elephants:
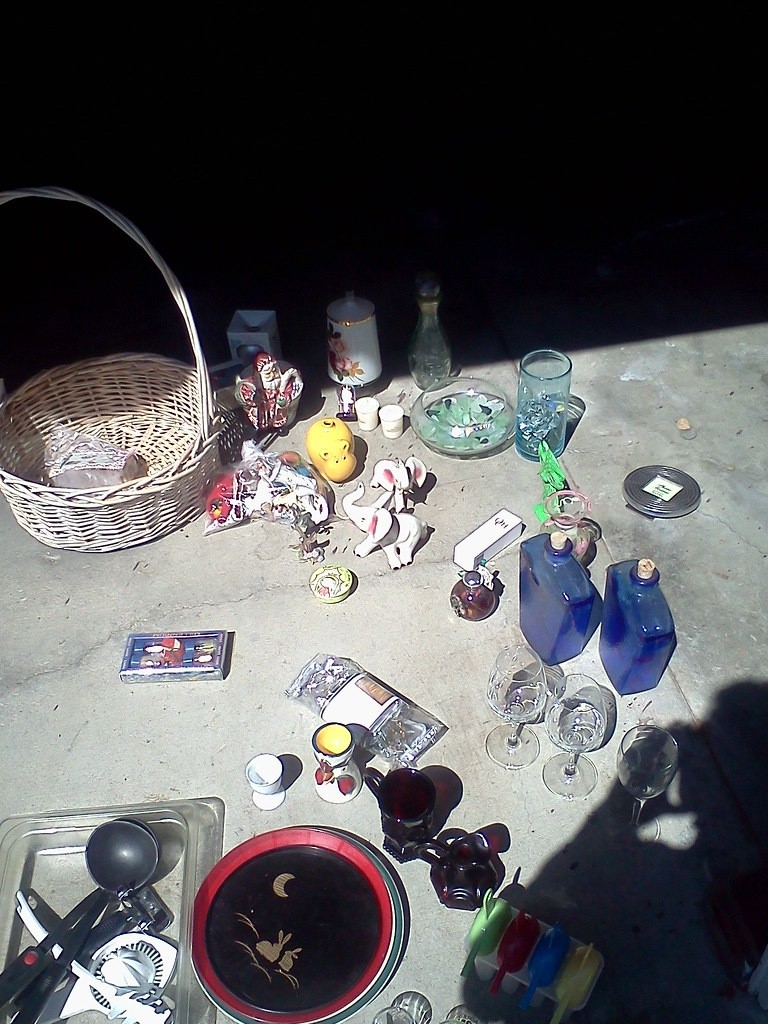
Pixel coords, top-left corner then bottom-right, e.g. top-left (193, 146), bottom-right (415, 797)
top-left (332, 457), bottom-right (428, 570)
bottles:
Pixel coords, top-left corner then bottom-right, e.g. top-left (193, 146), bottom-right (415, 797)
top-left (518, 531), bottom-right (594, 667)
top-left (408, 281), bottom-right (452, 390)
top-left (599, 558), bottom-right (674, 696)
top-left (540, 491), bottom-right (590, 563)
top-left (449, 571), bottom-right (495, 621)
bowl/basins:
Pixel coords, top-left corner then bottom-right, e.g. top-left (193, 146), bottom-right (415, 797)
top-left (410, 377), bottom-right (515, 455)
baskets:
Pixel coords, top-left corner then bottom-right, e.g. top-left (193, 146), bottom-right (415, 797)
top-left (0, 182), bottom-right (223, 553)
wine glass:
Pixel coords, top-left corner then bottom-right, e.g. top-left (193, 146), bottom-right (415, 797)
top-left (542, 673), bottom-right (607, 797)
top-left (485, 644), bottom-right (547, 769)
top-left (616, 725), bottom-right (678, 847)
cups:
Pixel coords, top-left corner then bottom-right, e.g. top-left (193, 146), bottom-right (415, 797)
top-left (245, 753), bottom-right (283, 795)
top-left (379, 404), bottom-right (405, 439)
top-left (327, 291), bottom-right (382, 388)
top-left (373, 1007), bottom-right (415, 1024)
top-left (515, 349), bottom-right (572, 461)
top-left (440, 1020), bottom-right (463, 1024)
top-left (410, 833), bottom-right (496, 911)
top-left (362, 767), bottom-right (438, 864)
top-left (355, 397), bottom-right (379, 430)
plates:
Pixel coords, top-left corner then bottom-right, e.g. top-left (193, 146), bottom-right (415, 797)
top-left (187, 825), bottom-right (404, 1024)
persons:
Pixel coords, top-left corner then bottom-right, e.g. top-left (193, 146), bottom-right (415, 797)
top-left (237, 353), bottom-right (298, 437)
